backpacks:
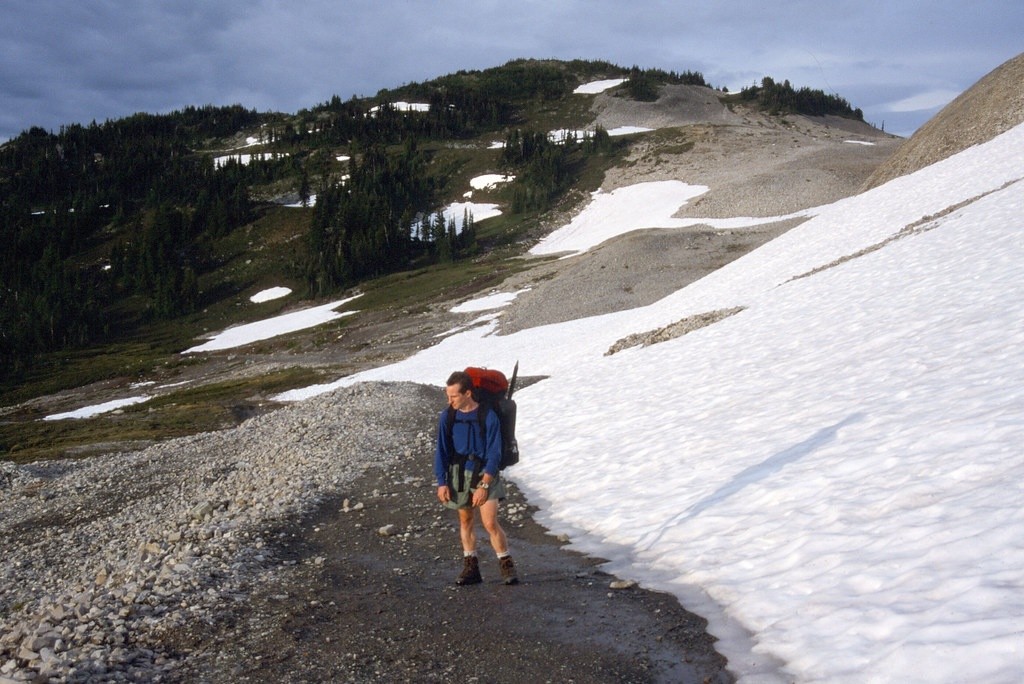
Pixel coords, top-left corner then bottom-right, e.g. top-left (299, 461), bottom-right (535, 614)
top-left (447, 367), bottom-right (519, 489)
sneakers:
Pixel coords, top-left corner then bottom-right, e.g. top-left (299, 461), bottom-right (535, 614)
top-left (499, 555), bottom-right (520, 585)
top-left (455, 556), bottom-right (483, 584)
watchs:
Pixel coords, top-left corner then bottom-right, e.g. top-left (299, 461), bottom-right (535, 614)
top-left (478, 481), bottom-right (490, 490)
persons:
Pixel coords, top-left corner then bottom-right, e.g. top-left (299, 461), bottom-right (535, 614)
top-left (433, 370), bottom-right (519, 589)
